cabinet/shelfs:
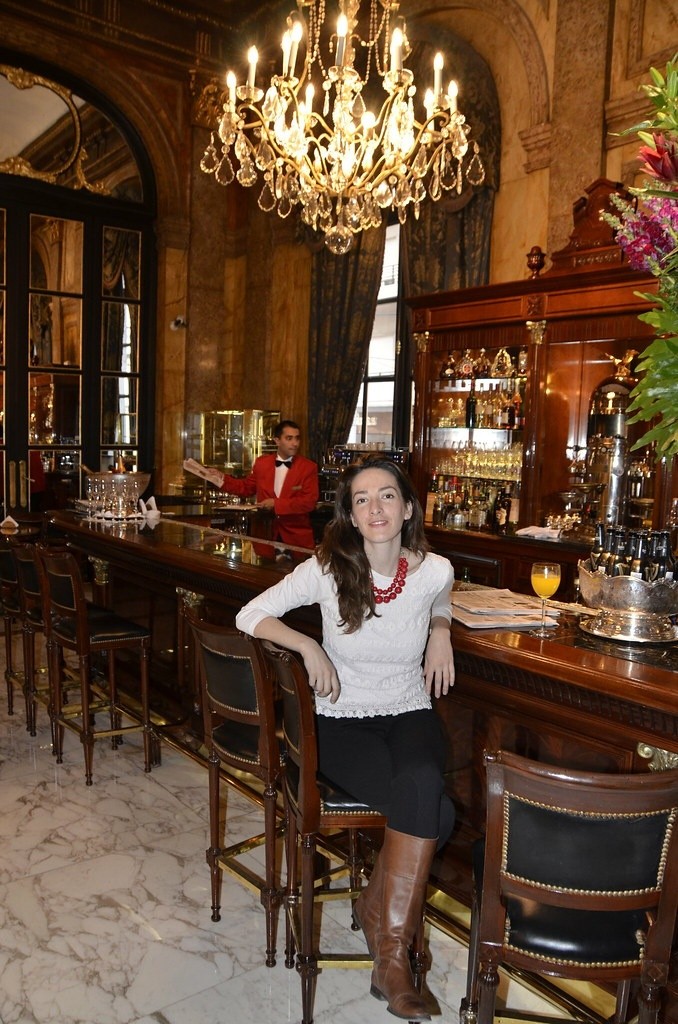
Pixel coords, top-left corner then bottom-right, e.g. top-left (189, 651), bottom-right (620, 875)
top-left (402, 259), bottom-right (678, 603)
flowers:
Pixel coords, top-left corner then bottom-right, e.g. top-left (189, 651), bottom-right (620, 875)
top-left (594, 55), bottom-right (677, 455)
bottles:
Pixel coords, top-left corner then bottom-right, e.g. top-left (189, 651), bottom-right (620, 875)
top-left (459, 568), bottom-right (471, 584)
top-left (590, 521), bottom-right (676, 586)
top-left (428, 348), bottom-right (531, 534)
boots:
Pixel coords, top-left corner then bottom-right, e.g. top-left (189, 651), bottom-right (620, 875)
top-left (370, 823), bottom-right (441, 1021)
top-left (353, 843), bottom-right (387, 961)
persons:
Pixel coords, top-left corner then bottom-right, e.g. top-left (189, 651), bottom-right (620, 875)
top-left (207, 420), bottom-right (318, 513)
top-left (235, 454), bottom-right (456, 1024)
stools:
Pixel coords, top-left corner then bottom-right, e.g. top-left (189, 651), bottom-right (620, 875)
top-left (1, 527), bottom-right (678, 1023)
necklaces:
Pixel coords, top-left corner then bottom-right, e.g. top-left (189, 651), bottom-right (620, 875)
top-left (370, 547), bottom-right (406, 603)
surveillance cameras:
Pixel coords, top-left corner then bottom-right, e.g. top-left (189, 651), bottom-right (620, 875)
top-left (170, 318), bottom-right (183, 331)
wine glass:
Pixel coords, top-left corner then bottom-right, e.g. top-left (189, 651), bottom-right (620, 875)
top-left (558, 481), bottom-right (654, 533)
top-left (85, 476), bottom-right (140, 523)
top-left (531, 561), bottom-right (561, 638)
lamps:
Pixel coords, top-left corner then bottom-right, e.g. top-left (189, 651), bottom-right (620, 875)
top-left (186, 0), bottom-right (485, 256)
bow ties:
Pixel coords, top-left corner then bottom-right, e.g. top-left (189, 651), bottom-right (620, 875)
top-left (275, 460), bottom-right (293, 468)
